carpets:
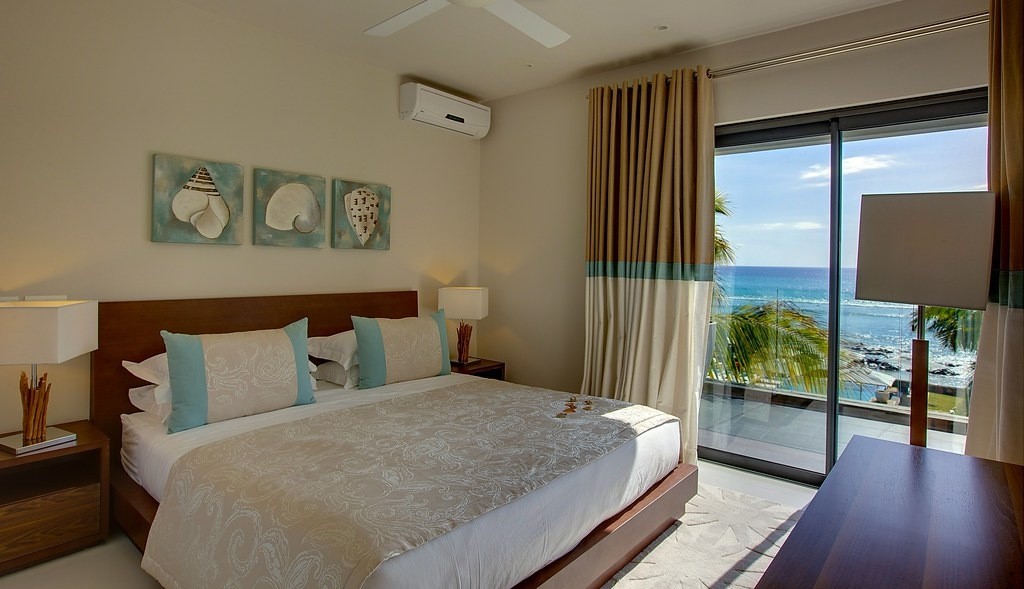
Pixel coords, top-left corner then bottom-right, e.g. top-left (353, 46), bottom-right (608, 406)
top-left (598, 483), bottom-right (807, 589)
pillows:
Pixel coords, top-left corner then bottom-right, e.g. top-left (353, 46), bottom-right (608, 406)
top-left (307, 309), bottom-right (452, 391)
top-left (122, 314), bottom-right (317, 435)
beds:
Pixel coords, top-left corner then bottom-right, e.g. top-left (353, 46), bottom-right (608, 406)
top-left (89, 290), bottom-right (698, 589)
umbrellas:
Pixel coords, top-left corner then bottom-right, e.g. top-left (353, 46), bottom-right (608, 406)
top-left (757, 358), bottom-right (812, 376)
top-left (842, 366), bottom-right (895, 399)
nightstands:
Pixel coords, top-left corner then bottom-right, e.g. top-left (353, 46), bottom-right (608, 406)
top-left (0, 419), bottom-right (111, 577)
top-left (449, 356), bottom-right (506, 381)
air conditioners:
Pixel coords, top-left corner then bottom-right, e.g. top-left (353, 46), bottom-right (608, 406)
top-left (399, 82), bottom-right (496, 140)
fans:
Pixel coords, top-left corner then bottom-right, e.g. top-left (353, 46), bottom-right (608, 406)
top-left (363, 0), bottom-right (573, 48)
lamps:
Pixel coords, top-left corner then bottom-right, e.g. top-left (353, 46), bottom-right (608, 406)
top-left (438, 286), bottom-right (489, 368)
top-left (0, 299), bottom-right (99, 456)
top-left (855, 190), bottom-right (999, 449)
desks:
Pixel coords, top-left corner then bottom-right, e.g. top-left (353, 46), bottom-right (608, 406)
top-left (753, 435), bottom-right (1024, 589)
